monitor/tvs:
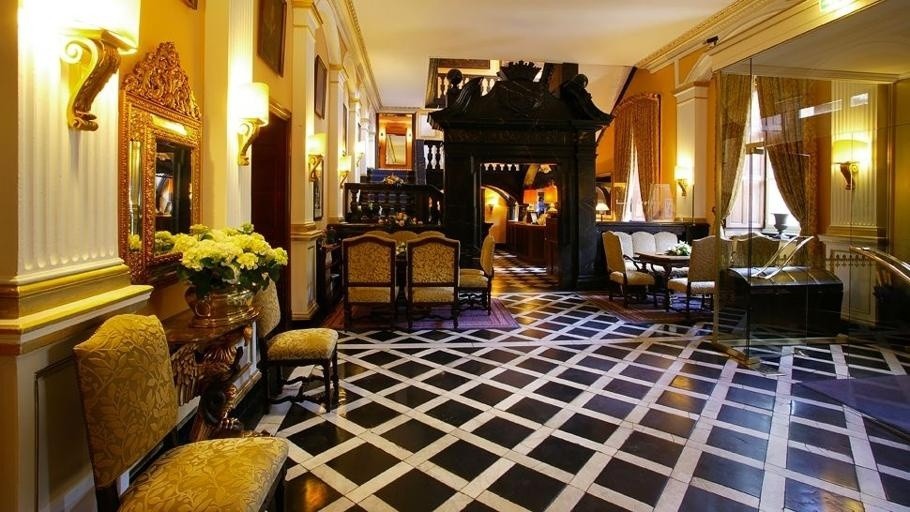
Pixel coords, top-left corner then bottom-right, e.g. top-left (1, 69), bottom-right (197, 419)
top-left (528, 211), bottom-right (539, 223)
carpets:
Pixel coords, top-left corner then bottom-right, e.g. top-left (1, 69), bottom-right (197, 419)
top-left (319, 297), bottom-right (521, 331)
top-left (797, 372), bottom-right (909, 443)
top-left (573, 290), bottom-right (714, 325)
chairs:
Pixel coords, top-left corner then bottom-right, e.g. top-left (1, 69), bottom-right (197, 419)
top-left (340, 229), bottom-right (496, 330)
top-left (251, 272), bottom-right (340, 415)
top-left (70, 312), bottom-right (291, 512)
top-left (601, 230), bottom-right (825, 324)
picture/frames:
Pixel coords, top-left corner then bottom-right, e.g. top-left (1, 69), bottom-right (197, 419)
top-left (314, 54), bottom-right (327, 120)
top-left (344, 104), bottom-right (349, 153)
top-left (313, 158), bottom-right (325, 221)
top-left (184, 0), bottom-right (198, 9)
top-left (257, 0), bottom-right (288, 78)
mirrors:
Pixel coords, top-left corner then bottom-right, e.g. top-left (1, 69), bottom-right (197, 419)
top-left (117, 39), bottom-right (204, 288)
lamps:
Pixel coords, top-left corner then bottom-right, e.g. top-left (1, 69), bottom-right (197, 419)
top-left (834, 139), bottom-right (869, 191)
top-left (238, 81), bottom-right (271, 167)
top-left (613, 182), bottom-right (628, 205)
top-left (675, 165), bottom-right (690, 197)
top-left (308, 133), bottom-right (325, 182)
top-left (339, 155), bottom-right (352, 188)
top-left (488, 203), bottom-right (495, 214)
top-left (61, 0), bottom-right (144, 128)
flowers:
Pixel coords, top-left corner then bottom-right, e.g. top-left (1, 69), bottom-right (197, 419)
top-left (378, 212), bottom-right (425, 228)
top-left (381, 173), bottom-right (408, 187)
top-left (171, 222), bottom-right (289, 299)
top-left (128, 229), bottom-right (180, 255)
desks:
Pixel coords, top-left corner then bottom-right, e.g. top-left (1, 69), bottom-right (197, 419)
top-left (160, 308), bottom-right (274, 442)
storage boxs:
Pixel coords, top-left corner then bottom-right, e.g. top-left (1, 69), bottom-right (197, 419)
top-left (717, 266), bottom-right (844, 339)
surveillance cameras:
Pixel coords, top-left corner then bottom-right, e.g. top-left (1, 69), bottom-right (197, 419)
top-left (703, 35), bottom-right (719, 48)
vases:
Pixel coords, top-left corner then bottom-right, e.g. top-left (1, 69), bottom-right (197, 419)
top-left (184, 284), bottom-right (260, 329)
